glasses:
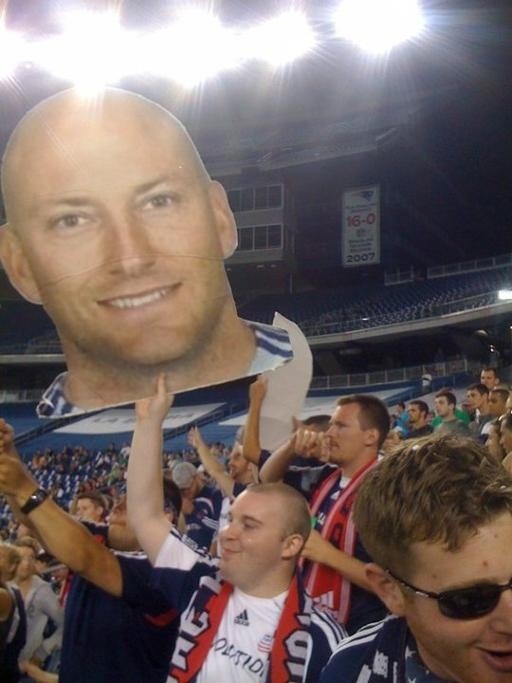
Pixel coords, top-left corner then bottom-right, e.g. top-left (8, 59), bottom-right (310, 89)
top-left (386, 570), bottom-right (512, 622)
top-left (487, 398), bottom-right (497, 403)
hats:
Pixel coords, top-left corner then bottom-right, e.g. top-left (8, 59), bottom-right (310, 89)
top-left (173, 462), bottom-right (198, 491)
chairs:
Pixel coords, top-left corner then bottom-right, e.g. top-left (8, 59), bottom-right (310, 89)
top-left (0, 376), bottom-right (421, 508)
top-left (236, 266), bottom-right (512, 348)
top-left (1, 298), bottom-right (61, 354)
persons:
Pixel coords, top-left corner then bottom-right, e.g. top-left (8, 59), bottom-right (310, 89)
top-left (2, 323), bottom-right (512, 683)
top-left (0, 83), bottom-right (295, 419)
